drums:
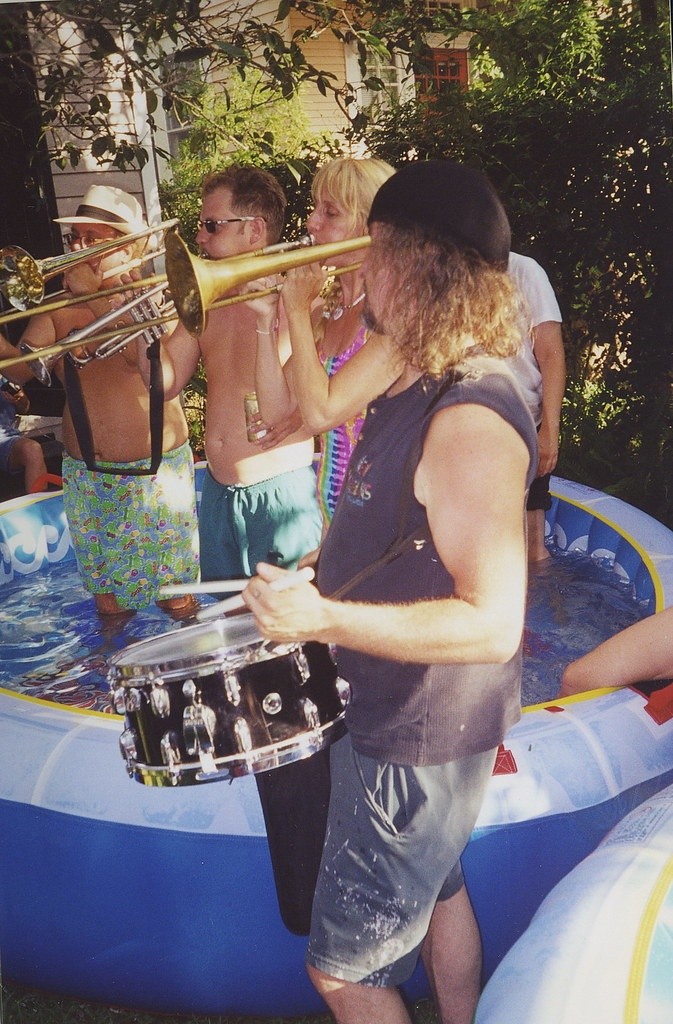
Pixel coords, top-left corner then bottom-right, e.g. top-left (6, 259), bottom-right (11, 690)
top-left (105, 612), bottom-right (352, 787)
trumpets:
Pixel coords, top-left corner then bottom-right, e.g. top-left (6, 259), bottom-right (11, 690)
top-left (1, 216), bottom-right (375, 386)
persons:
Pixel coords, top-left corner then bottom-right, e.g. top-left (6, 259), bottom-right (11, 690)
top-left (0, 185), bottom-right (202, 613)
top-left (104, 160), bottom-right (328, 620)
top-left (243, 159), bottom-right (397, 534)
top-left (505, 252), bottom-right (566, 561)
top-left (243, 158), bottom-right (541, 1024)
top-left (0, 374), bottom-right (48, 496)
top-left (560, 607), bottom-right (673, 695)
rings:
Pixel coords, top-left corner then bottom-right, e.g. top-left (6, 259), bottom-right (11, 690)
top-left (254, 584), bottom-right (267, 598)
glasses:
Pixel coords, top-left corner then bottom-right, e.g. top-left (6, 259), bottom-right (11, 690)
top-left (64, 234), bottom-right (115, 251)
top-left (197, 217), bottom-right (268, 234)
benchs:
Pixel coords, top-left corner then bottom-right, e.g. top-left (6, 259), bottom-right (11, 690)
top-left (0, 440), bottom-right (64, 503)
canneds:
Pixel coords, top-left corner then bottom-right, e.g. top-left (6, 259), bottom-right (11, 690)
top-left (245, 392), bottom-right (271, 441)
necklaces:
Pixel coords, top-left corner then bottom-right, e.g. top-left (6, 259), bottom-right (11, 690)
top-left (332, 292), bottom-right (366, 321)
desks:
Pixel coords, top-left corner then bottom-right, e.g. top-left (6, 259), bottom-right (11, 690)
top-left (16, 414), bottom-right (63, 444)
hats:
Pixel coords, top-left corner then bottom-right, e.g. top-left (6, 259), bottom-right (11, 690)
top-left (366, 161), bottom-right (511, 272)
top-left (53, 184), bottom-right (157, 252)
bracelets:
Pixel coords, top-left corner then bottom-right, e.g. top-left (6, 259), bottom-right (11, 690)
top-left (256, 330), bottom-right (271, 334)
